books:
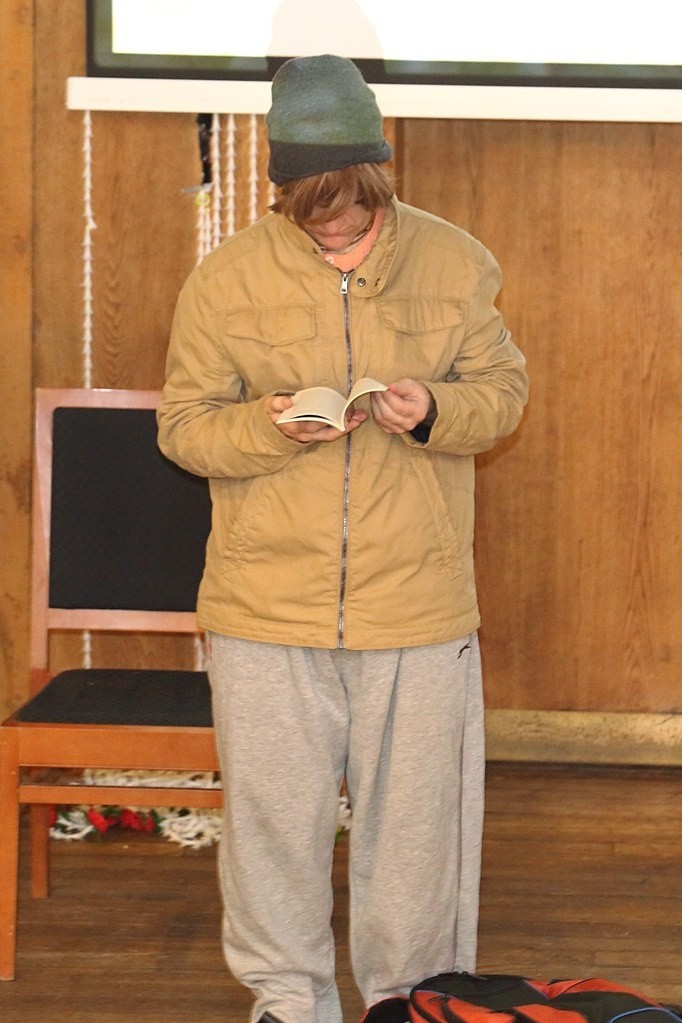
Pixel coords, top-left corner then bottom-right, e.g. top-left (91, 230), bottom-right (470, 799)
top-left (275, 376), bottom-right (390, 432)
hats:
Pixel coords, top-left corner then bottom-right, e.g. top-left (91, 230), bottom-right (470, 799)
top-left (265, 54), bottom-right (393, 184)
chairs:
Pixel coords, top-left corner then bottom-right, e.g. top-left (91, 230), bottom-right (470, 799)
top-left (0, 390), bottom-right (224, 981)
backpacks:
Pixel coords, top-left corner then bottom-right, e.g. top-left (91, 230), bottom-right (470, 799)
top-left (356, 970), bottom-right (682, 1023)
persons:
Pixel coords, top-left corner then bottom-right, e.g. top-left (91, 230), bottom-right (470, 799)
top-left (156, 54), bottom-right (529, 1023)
